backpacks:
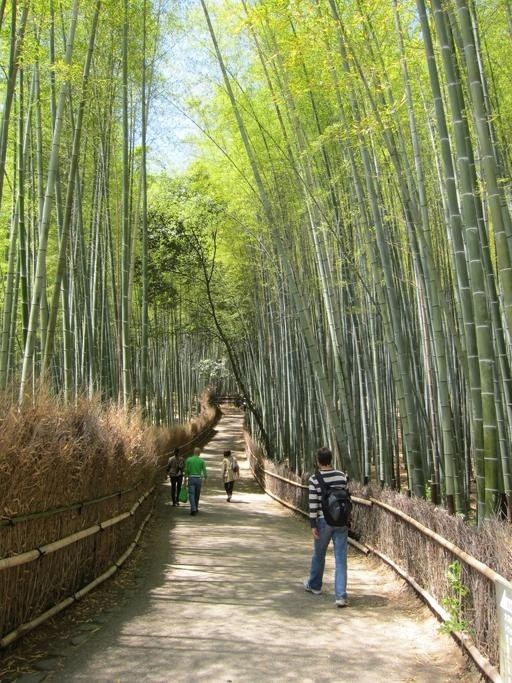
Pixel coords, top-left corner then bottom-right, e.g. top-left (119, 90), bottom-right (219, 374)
top-left (314, 469), bottom-right (353, 528)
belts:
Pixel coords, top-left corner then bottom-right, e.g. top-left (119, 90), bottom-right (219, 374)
top-left (188, 474), bottom-right (201, 478)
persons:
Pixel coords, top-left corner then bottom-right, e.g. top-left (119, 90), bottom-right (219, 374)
top-left (303, 445), bottom-right (351, 608)
top-left (184, 446), bottom-right (208, 515)
top-left (221, 448), bottom-right (239, 502)
top-left (164, 447), bottom-right (185, 507)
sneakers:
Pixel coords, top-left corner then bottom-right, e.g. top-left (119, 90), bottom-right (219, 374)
top-left (190, 509), bottom-right (199, 515)
top-left (334, 597), bottom-right (349, 607)
top-left (227, 495), bottom-right (232, 502)
top-left (302, 578), bottom-right (323, 594)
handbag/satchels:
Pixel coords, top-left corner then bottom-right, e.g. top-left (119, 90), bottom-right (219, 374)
top-left (178, 476), bottom-right (188, 503)
top-left (231, 457), bottom-right (239, 473)
